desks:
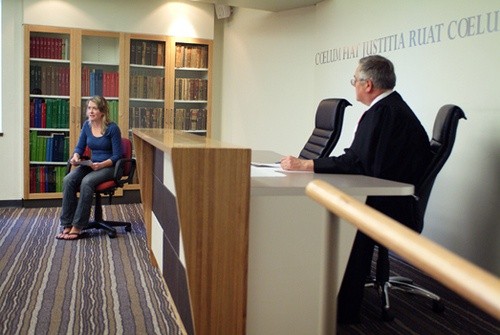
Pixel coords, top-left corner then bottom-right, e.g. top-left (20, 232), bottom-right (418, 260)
top-left (245, 151), bottom-right (414, 335)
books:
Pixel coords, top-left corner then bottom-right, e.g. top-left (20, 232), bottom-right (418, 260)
top-left (29, 36), bottom-right (70, 193)
top-left (81, 65), bottom-right (119, 126)
top-left (175, 44), bottom-right (208, 130)
top-left (129, 41), bottom-right (164, 158)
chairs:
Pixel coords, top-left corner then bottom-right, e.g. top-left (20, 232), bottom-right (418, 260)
top-left (365, 104), bottom-right (468, 324)
top-left (66, 138), bottom-right (136, 239)
top-left (296, 98), bottom-right (352, 160)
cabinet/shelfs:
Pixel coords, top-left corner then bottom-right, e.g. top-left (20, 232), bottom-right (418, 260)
top-left (22, 23), bottom-right (214, 199)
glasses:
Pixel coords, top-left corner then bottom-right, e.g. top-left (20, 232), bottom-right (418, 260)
top-left (350, 78), bottom-right (367, 87)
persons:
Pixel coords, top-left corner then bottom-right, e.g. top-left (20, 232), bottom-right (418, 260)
top-left (56, 95), bottom-right (125, 241)
top-left (280, 55), bottom-right (430, 323)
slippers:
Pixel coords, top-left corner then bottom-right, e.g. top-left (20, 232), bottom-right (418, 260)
top-left (55, 229), bottom-right (70, 240)
top-left (66, 231), bottom-right (81, 241)
